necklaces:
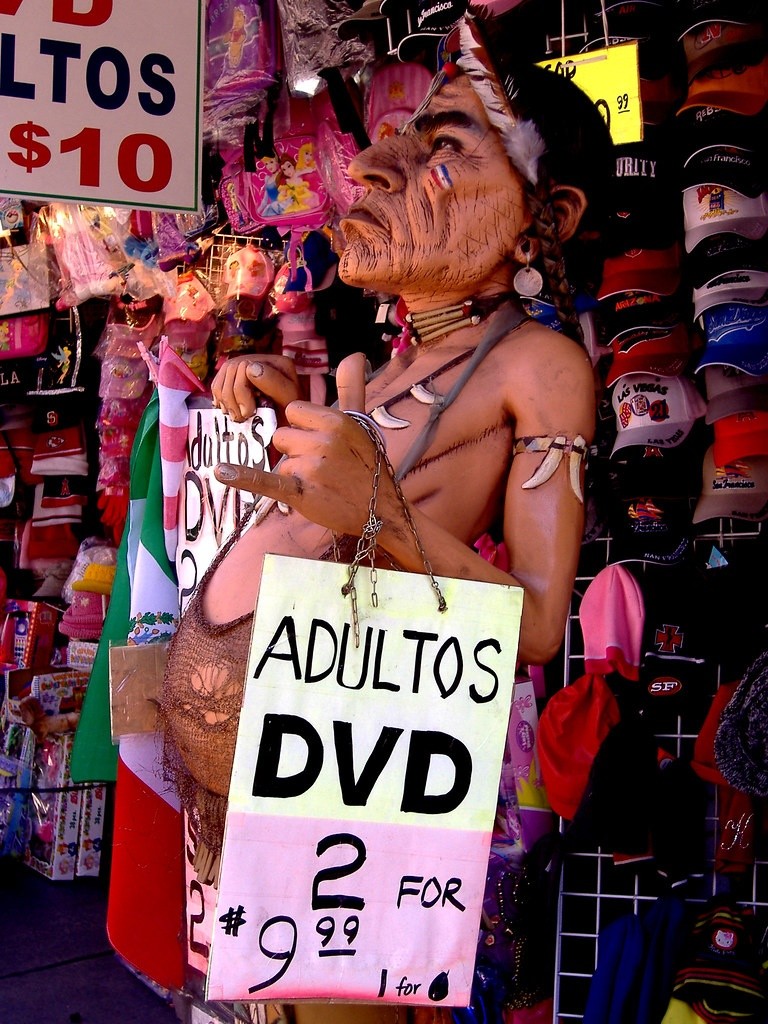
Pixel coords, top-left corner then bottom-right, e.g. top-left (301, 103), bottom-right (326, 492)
top-left (407, 297), bottom-right (486, 349)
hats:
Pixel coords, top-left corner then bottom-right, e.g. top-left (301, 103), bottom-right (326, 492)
top-left (342, 0), bottom-right (767, 1023)
top-left (0, 202), bottom-right (326, 640)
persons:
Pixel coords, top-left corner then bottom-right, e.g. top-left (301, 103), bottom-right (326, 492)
top-left (160, 57), bottom-right (616, 1024)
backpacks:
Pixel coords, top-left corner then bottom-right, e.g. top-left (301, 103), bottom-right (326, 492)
top-left (203, 0), bottom-right (277, 104)
top-left (218, 84), bottom-right (367, 234)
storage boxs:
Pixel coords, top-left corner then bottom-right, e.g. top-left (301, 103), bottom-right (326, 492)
top-left (0, 601), bottom-right (106, 881)
